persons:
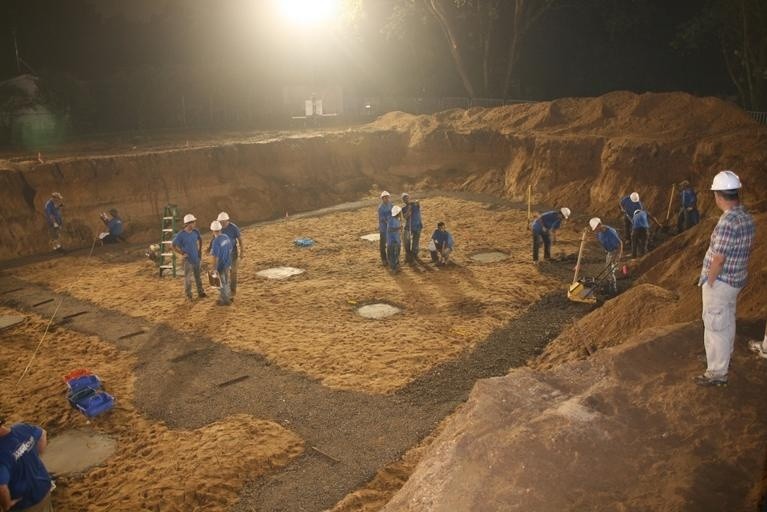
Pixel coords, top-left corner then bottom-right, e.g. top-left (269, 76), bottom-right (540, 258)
top-left (630, 209), bottom-right (663, 259)
top-left (204, 211), bottom-right (245, 296)
top-left (207, 220), bottom-right (232, 306)
top-left (171, 213), bottom-right (209, 302)
top-left (590, 217), bottom-right (623, 298)
top-left (530, 207), bottom-right (571, 266)
top-left (428, 221), bottom-right (454, 267)
top-left (618, 192), bottom-right (642, 249)
top-left (691, 170), bottom-right (756, 388)
top-left (747, 322), bottom-right (767, 357)
top-left (45, 192), bottom-right (65, 257)
top-left (376, 190), bottom-right (424, 275)
top-left (0, 417), bottom-right (53, 511)
top-left (96, 208), bottom-right (123, 245)
top-left (676, 180), bottom-right (697, 234)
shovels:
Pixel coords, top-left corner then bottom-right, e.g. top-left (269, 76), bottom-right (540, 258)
top-left (406, 209), bottom-right (415, 267)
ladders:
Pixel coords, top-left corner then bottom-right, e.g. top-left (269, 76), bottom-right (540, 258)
top-left (158, 207), bottom-right (176, 278)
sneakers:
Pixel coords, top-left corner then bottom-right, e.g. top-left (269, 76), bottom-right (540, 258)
top-left (748, 339), bottom-right (767, 358)
top-left (692, 375), bottom-right (727, 388)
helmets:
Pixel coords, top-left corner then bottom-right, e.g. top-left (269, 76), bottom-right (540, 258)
top-left (590, 218), bottom-right (601, 231)
top-left (217, 212), bottom-right (229, 221)
top-left (630, 192), bottom-right (639, 202)
top-left (381, 191), bottom-right (389, 198)
top-left (561, 208), bottom-right (570, 219)
top-left (184, 214), bottom-right (196, 223)
top-left (710, 171), bottom-right (741, 190)
top-left (210, 221), bottom-right (222, 231)
top-left (391, 206), bottom-right (401, 216)
top-left (402, 193), bottom-right (408, 199)
top-left (429, 241), bottom-right (436, 251)
